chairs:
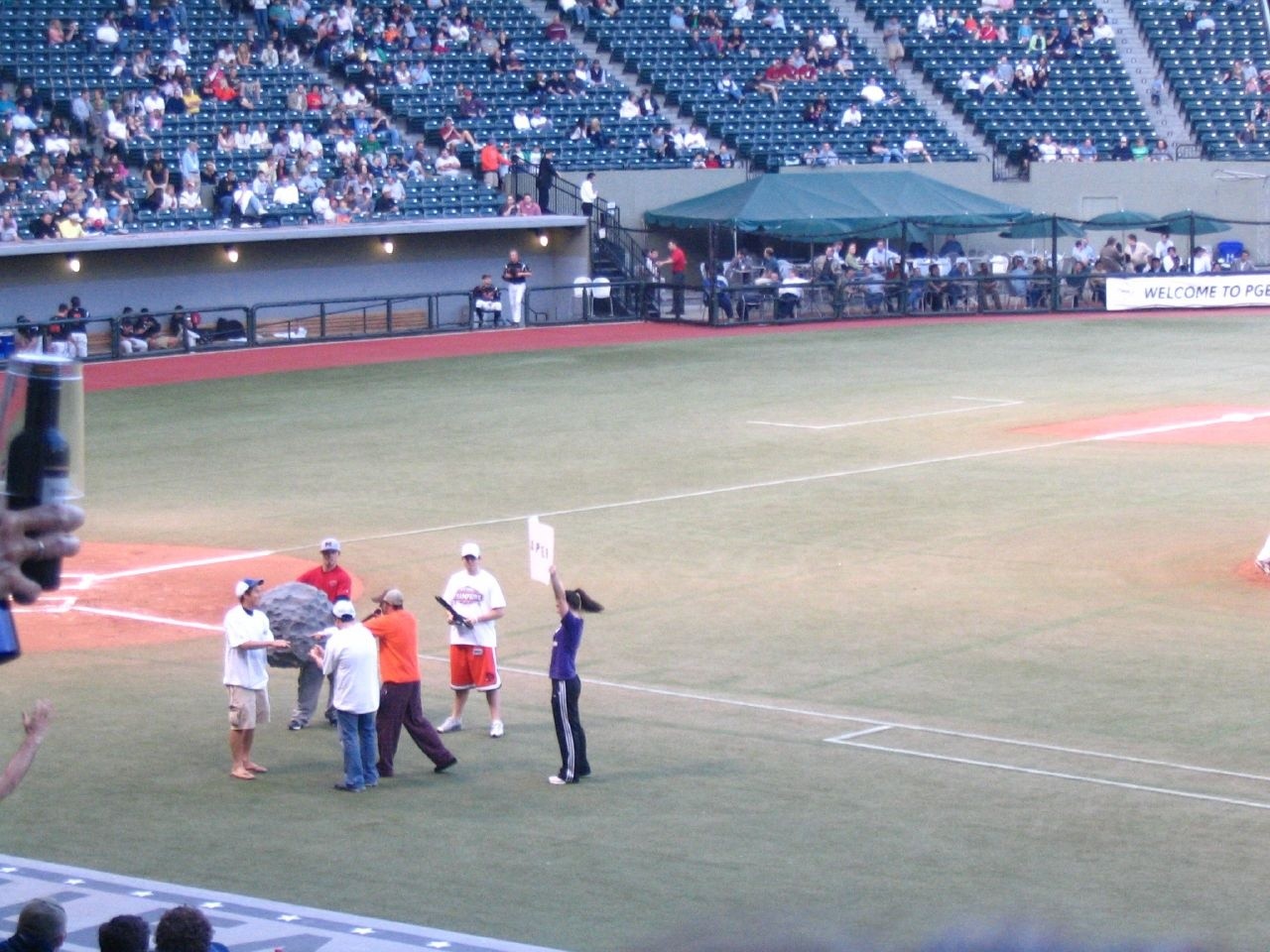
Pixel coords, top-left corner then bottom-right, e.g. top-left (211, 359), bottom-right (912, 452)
top-left (572, 277), bottom-right (613, 317)
top-left (741, 291), bottom-right (765, 320)
top-left (0, 0), bottom-right (1270, 238)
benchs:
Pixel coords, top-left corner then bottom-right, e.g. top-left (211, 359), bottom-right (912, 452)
top-left (87, 309), bottom-right (427, 354)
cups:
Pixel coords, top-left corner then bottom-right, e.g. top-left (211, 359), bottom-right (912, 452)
top-left (0, 353), bottom-right (86, 501)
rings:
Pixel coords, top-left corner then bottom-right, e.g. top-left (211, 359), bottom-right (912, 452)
top-left (35, 542), bottom-right (48, 561)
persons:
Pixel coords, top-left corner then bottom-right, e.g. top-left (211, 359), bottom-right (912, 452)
top-left (306, 601), bottom-right (380, 791)
top-left (0, 0), bottom-right (1270, 362)
top-left (0, 482), bottom-right (86, 604)
top-left (436, 543), bottom-right (507, 737)
top-left (222, 578), bottom-right (292, 780)
top-left (151, 902), bottom-right (229, 952)
top-left (98, 914), bottom-right (151, 952)
top-left (549, 567), bottom-right (607, 786)
top-left (288, 536), bottom-right (353, 730)
top-left (0, 697), bottom-right (56, 801)
top-left (360, 590), bottom-right (457, 778)
top-left (0, 896), bottom-right (68, 952)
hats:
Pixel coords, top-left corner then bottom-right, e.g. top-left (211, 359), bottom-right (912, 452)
top-left (68, 214), bottom-right (84, 222)
top-left (332, 600), bottom-right (355, 620)
top-left (371, 589), bottom-right (404, 606)
top-left (320, 539), bottom-right (340, 554)
top-left (235, 579), bottom-right (264, 600)
top-left (462, 543), bottom-right (481, 559)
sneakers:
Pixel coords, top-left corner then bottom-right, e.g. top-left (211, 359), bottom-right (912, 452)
top-left (549, 776), bottom-right (566, 784)
top-left (436, 717), bottom-right (462, 733)
top-left (490, 720), bottom-right (503, 736)
top-left (288, 718), bottom-right (308, 730)
top-left (325, 710), bottom-right (337, 727)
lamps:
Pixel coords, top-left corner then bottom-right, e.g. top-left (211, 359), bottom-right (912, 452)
top-left (66, 252), bottom-right (80, 271)
top-left (224, 245), bottom-right (238, 264)
top-left (535, 229), bottom-right (548, 247)
top-left (379, 235), bottom-right (393, 254)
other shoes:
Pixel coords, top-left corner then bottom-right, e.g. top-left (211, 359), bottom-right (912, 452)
top-left (433, 758), bottom-right (457, 772)
top-left (335, 783), bottom-right (359, 793)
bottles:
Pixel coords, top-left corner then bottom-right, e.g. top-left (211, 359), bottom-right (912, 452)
top-left (0, 360), bottom-right (74, 590)
